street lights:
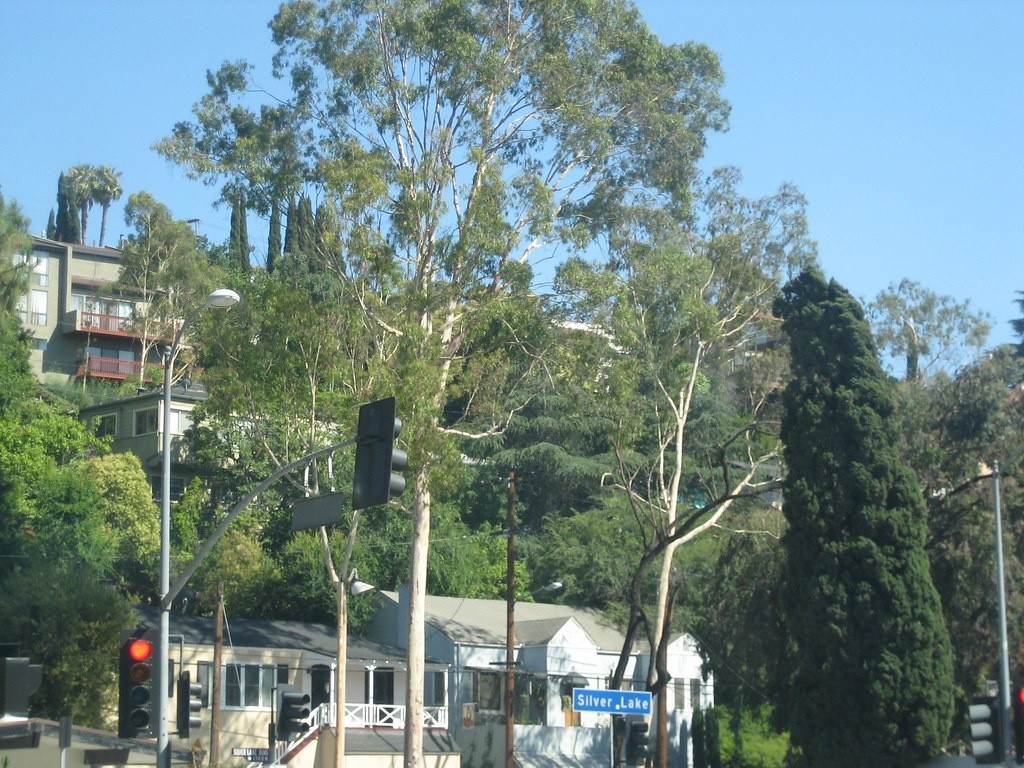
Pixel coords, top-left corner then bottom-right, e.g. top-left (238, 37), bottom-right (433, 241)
top-left (154, 288), bottom-right (241, 766)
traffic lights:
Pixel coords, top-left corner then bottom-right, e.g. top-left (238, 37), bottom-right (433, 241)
top-left (352, 396), bottom-right (409, 510)
top-left (274, 683), bottom-right (310, 743)
top-left (1012, 675), bottom-right (1024, 766)
top-left (176, 669), bottom-right (204, 739)
top-left (609, 714), bottom-right (650, 768)
top-left (964, 695), bottom-right (1005, 766)
top-left (117, 628), bottom-right (160, 740)
